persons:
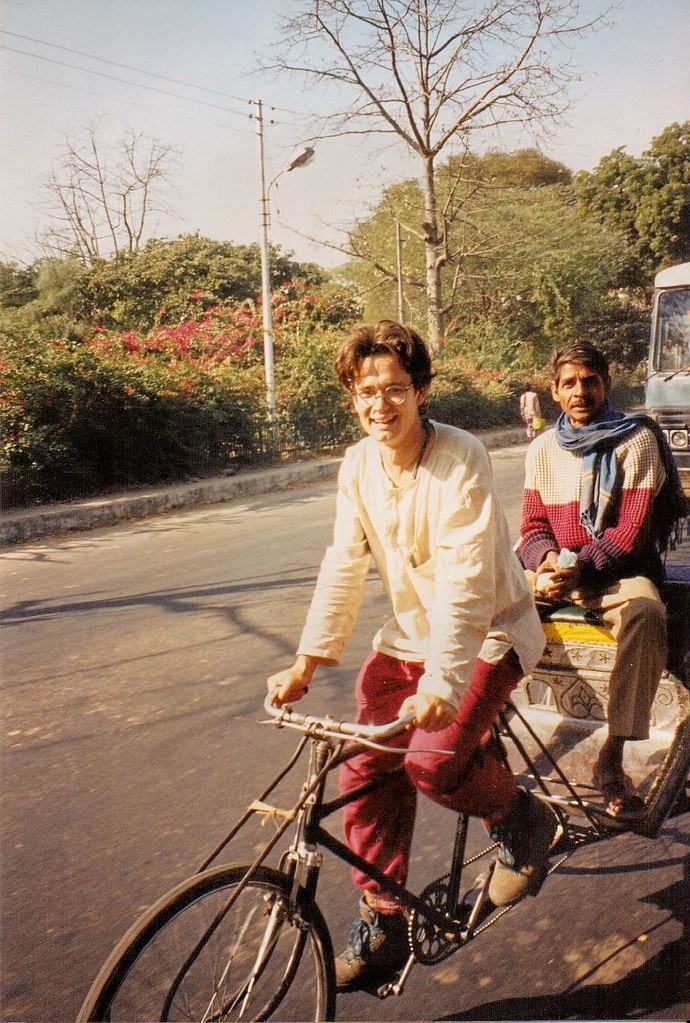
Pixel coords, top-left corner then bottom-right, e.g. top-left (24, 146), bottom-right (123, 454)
top-left (481, 341), bottom-right (669, 815)
top-left (520, 384), bottom-right (542, 441)
top-left (267, 318), bottom-right (558, 992)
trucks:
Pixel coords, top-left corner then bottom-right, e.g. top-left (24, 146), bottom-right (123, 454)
top-left (642, 262), bottom-right (690, 502)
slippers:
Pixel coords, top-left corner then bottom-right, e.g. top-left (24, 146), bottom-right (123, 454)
top-left (591, 775), bottom-right (646, 819)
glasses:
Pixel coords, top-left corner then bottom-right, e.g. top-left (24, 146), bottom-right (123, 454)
top-left (350, 384), bottom-right (413, 407)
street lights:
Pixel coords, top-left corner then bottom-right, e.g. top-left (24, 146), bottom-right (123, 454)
top-left (257, 147), bottom-right (316, 426)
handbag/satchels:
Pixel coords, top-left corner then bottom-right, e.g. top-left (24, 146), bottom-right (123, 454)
top-left (532, 416), bottom-right (545, 431)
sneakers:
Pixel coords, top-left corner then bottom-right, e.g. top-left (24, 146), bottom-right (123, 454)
top-left (335, 894), bottom-right (416, 993)
top-left (481, 791), bottom-right (557, 906)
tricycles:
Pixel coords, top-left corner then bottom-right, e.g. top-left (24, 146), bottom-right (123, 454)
top-left (72, 583), bottom-right (690, 1023)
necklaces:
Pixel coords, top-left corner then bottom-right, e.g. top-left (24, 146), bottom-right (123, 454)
top-left (413, 421), bottom-right (431, 480)
